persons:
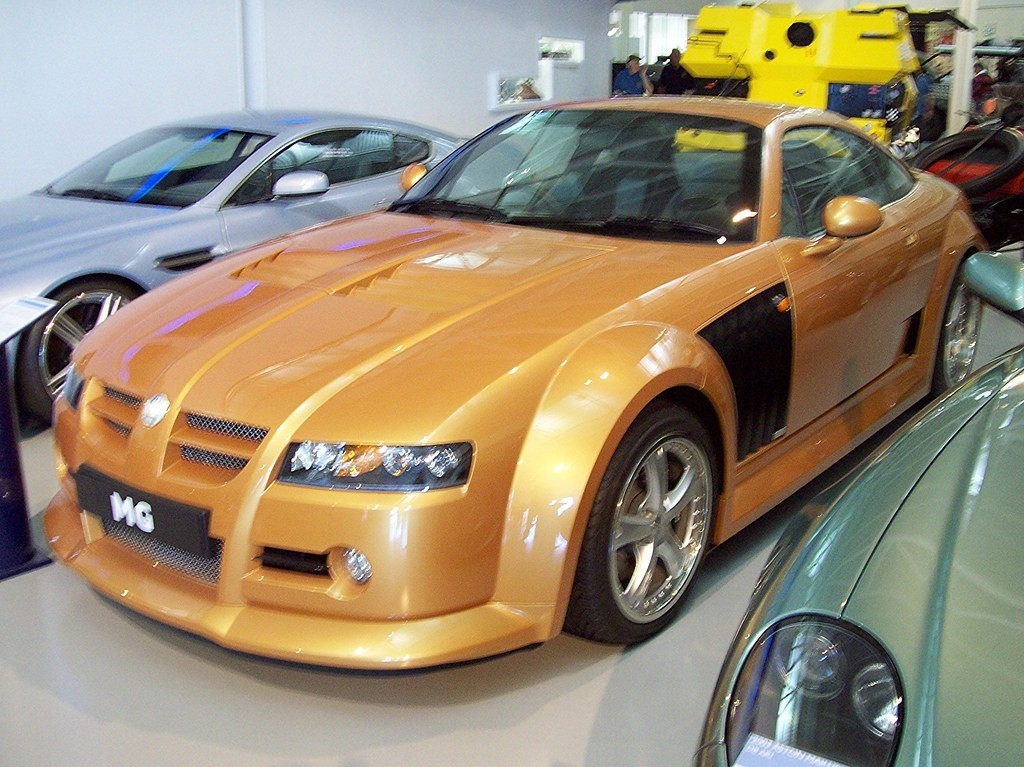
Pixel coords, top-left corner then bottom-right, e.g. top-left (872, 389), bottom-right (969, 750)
top-left (614, 55), bottom-right (654, 94)
top-left (915, 62), bottom-right (998, 141)
top-left (656, 49), bottom-right (715, 95)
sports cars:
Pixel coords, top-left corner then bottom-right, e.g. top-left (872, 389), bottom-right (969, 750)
top-left (40, 96), bottom-right (982, 672)
top-left (0, 102), bottom-right (574, 411)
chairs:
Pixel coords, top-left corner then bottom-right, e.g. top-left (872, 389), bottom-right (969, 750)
top-left (778, 139), bottom-right (843, 237)
top-left (564, 124), bottom-right (678, 222)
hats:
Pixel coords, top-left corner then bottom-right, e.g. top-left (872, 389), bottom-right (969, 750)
top-left (626, 55), bottom-right (641, 64)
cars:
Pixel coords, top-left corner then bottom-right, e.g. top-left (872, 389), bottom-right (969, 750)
top-left (695, 244), bottom-right (1023, 767)
top-left (977, 38), bottom-right (1024, 73)
